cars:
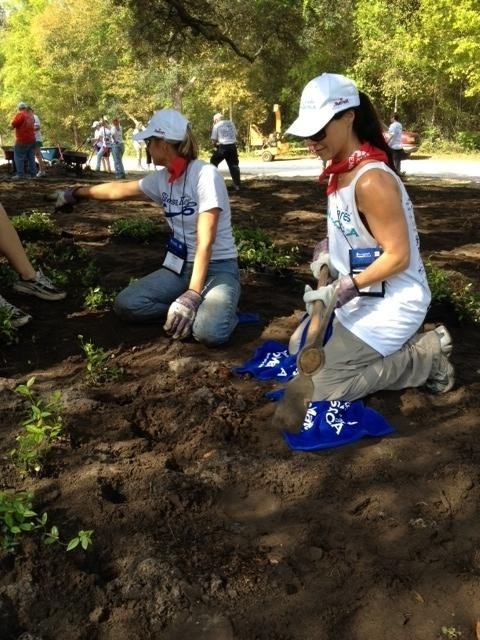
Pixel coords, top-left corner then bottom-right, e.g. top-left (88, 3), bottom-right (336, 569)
top-left (378, 120), bottom-right (419, 159)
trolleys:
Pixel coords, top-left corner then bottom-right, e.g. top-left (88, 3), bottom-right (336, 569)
top-left (2, 143), bottom-right (89, 168)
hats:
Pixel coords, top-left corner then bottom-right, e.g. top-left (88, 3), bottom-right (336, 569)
top-left (132, 108), bottom-right (192, 145)
top-left (283, 72), bottom-right (361, 138)
top-left (18, 102), bottom-right (27, 109)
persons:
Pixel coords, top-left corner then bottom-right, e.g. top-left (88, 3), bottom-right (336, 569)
top-left (211, 112), bottom-right (241, 191)
top-left (282, 72), bottom-right (457, 403)
top-left (385, 112), bottom-right (402, 170)
top-left (0, 203), bottom-right (67, 328)
top-left (7, 103), bottom-right (157, 178)
top-left (44, 108), bottom-right (241, 345)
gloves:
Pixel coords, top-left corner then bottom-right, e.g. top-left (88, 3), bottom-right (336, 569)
top-left (302, 273), bottom-right (361, 318)
top-left (53, 185), bottom-right (89, 215)
top-left (309, 237), bottom-right (340, 285)
top-left (162, 288), bottom-right (203, 340)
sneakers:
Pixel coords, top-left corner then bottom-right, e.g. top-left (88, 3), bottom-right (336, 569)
top-left (36, 170), bottom-right (46, 178)
top-left (11, 174), bottom-right (36, 181)
top-left (12, 268), bottom-right (68, 301)
top-left (0, 293), bottom-right (33, 329)
top-left (422, 322), bottom-right (456, 396)
